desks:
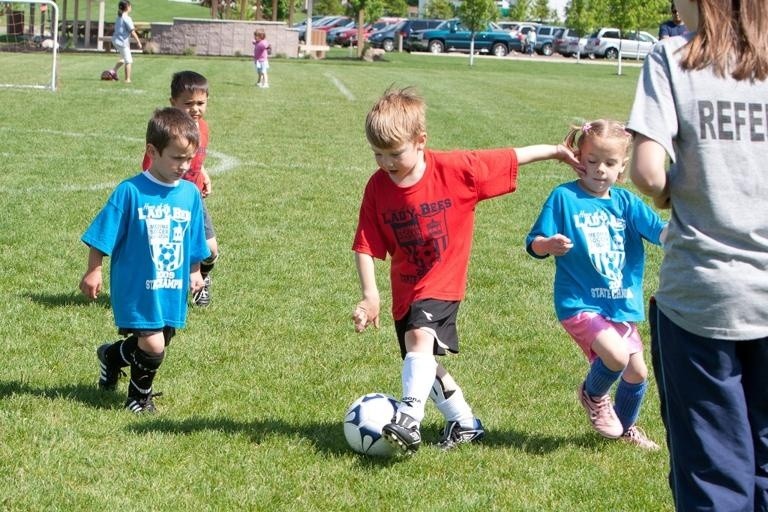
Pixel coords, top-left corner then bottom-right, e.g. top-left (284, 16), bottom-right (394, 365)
top-left (46, 18), bottom-right (151, 51)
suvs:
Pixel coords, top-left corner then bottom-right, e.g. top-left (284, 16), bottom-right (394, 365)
top-left (534, 25), bottom-right (565, 56)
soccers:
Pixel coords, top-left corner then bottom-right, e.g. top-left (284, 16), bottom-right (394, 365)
top-left (344, 392), bottom-right (407, 457)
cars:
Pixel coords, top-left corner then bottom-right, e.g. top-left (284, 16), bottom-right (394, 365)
top-left (292, 13), bottom-right (537, 63)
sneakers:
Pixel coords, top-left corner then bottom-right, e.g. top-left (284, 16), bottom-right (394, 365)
top-left (194, 274), bottom-right (211, 307)
top-left (125, 393), bottom-right (160, 416)
top-left (622, 424), bottom-right (661, 451)
top-left (577, 380), bottom-right (624, 440)
top-left (382, 421), bottom-right (421, 457)
top-left (114, 76), bottom-right (132, 83)
top-left (254, 81), bottom-right (269, 90)
top-left (96, 343), bottom-right (118, 391)
top-left (434, 419), bottom-right (487, 450)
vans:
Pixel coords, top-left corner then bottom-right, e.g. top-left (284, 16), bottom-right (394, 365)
top-left (550, 26), bottom-right (588, 57)
top-left (583, 27), bottom-right (659, 61)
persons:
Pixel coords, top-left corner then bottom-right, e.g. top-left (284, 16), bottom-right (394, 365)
top-left (80, 105), bottom-right (212, 422)
top-left (526, 26), bottom-right (537, 57)
top-left (111, 0), bottom-right (142, 83)
top-left (525, 120), bottom-right (668, 456)
top-left (658, 2), bottom-right (691, 38)
top-left (142, 72), bottom-right (220, 309)
top-left (252, 29), bottom-right (272, 88)
top-left (626, 1), bottom-right (767, 512)
top-left (351, 83), bottom-right (588, 453)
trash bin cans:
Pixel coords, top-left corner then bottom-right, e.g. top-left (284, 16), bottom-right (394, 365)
top-left (7, 11), bottom-right (25, 35)
top-left (312, 29), bottom-right (326, 58)
